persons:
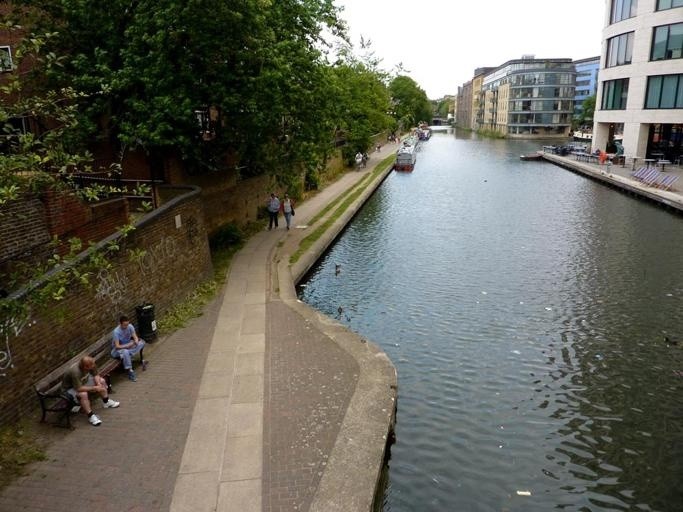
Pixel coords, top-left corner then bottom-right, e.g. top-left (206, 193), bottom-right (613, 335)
top-left (60, 356), bottom-right (119, 426)
top-left (280, 194), bottom-right (294, 230)
top-left (110, 316), bottom-right (148, 382)
top-left (267, 194), bottom-right (280, 230)
top-left (355, 134), bottom-right (396, 168)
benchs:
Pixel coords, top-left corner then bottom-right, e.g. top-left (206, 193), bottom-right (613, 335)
top-left (33, 333), bottom-right (143, 430)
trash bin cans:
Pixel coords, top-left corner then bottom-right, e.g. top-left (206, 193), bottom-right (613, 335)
top-left (619, 156), bottom-right (625, 165)
top-left (135, 303), bottom-right (157, 343)
top-left (560, 146), bottom-right (567, 155)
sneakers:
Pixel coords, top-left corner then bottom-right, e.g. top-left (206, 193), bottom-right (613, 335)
top-left (88, 413), bottom-right (102, 425)
top-left (129, 371), bottom-right (137, 381)
top-left (267, 226), bottom-right (290, 230)
top-left (104, 398), bottom-right (120, 408)
top-left (142, 360), bottom-right (149, 370)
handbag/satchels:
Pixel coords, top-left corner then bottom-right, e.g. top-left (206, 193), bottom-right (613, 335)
top-left (291, 204), bottom-right (295, 216)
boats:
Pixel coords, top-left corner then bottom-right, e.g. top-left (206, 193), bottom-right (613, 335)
top-left (519, 153), bottom-right (543, 160)
top-left (393, 128), bottom-right (432, 171)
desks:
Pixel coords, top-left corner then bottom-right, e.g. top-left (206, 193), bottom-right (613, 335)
top-left (619, 154), bottom-right (672, 172)
